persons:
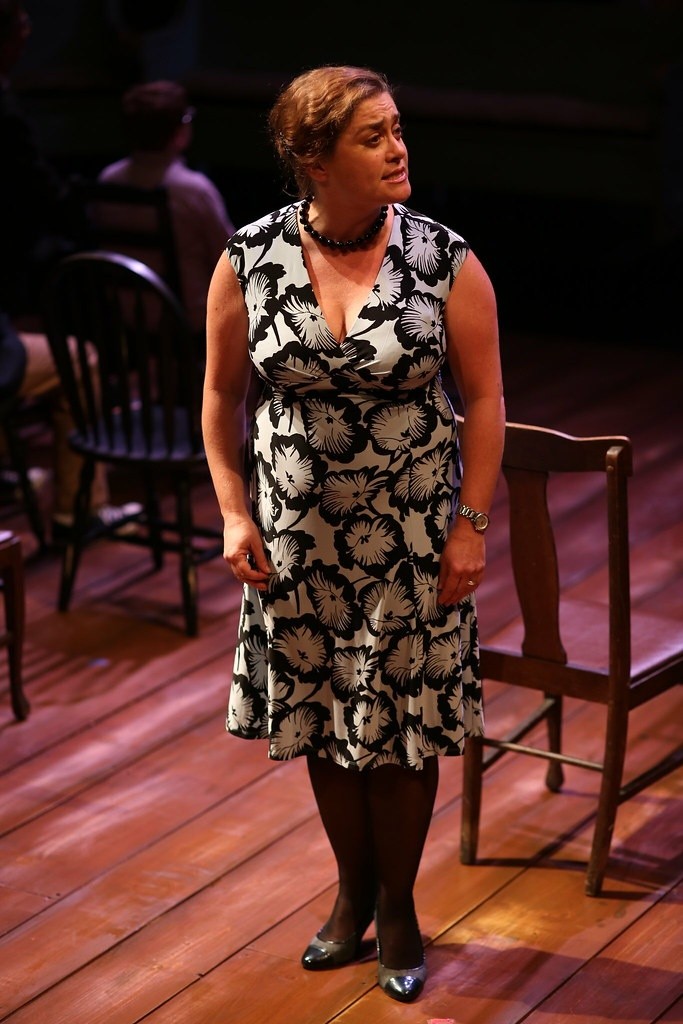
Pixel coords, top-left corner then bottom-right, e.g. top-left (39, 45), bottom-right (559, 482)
top-left (90, 80), bottom-right (235, 333)
top-left (199, 66), bottom-right (506, 1001)
top-left (0, 1), bottom-right (86, 299)
top-left (0, 312), bottom-right (143, 542)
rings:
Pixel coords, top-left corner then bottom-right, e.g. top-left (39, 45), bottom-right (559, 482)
top-left (468, 581), bottom-right (474, 586)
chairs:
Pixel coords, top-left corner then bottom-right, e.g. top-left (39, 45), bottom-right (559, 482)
top-left (36, 251), bottom-right (224, 637)
top-left (452, 412), bottom-right (683, 896)
top-left (0, 371), bottom-right (78, 564)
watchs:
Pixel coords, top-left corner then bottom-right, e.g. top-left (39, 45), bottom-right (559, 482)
top-left (454, 504), bottom-right (490, 534)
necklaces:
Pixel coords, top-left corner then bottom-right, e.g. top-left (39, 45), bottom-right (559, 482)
top-left (299, 194), bottom-right (389, 250)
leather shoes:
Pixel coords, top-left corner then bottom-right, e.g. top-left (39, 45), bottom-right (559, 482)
top-left (301, 900), bottom-right (427, 1003)
